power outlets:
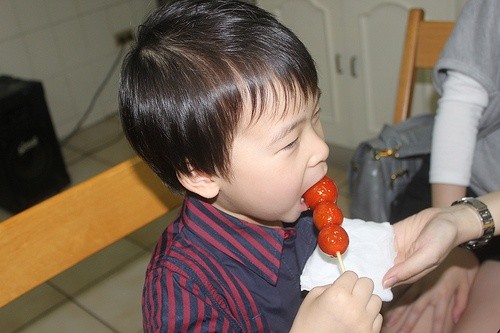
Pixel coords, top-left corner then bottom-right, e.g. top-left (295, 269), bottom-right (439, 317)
top-left (108, 21), bottom-right (139, 54)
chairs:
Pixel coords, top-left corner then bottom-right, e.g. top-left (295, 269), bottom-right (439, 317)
top-left (0, 156), bottom-right (184, 309)
top-left (393, 10), bottom-right (456, 123)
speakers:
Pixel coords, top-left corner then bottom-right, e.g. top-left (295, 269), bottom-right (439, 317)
top-left (0, 75), bottom-right (71, 214)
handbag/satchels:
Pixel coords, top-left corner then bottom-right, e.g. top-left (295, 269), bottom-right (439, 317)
top-left (389, 194), bottom-right (432, 230)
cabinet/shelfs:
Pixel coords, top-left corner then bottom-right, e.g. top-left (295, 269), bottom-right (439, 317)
top-left (255, 1), bottom-right (466, 151)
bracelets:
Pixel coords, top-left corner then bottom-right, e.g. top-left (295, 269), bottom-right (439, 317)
top-left (450, 196), bottom-right (495, 251)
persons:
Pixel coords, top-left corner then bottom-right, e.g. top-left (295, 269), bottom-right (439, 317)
top-left (119, 0), bottom-right (385, 332)
top-left (379, 0), bottom-right (500, 333)
